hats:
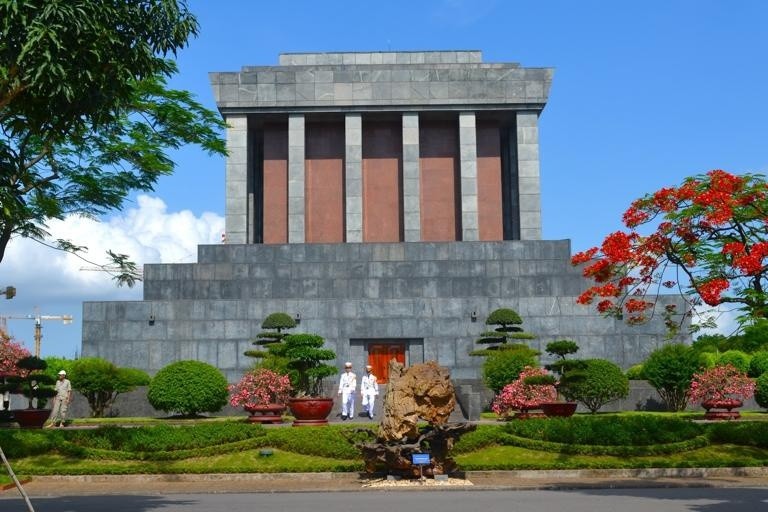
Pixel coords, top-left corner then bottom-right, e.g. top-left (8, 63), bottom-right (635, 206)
top-left (344, 363), bottom-right (351, 368)
top-left (366, 366), bottom-right (371, 372)
top-left (57, 370), bottom-right (68, 377)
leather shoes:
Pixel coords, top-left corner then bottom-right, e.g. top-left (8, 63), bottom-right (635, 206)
top-left (349, 415), bottom-right (352, 418)
top-left (369, 414), bottom-right (373, 418)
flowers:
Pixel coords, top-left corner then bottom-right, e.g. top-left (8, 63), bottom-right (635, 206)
top-left (491, 364), bottom-right (559, 416)
top-left (225, 367), bottom-right (295, 411)
top-left (685, 362), bottom-right (757, 408)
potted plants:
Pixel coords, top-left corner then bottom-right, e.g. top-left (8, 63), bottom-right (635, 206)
top-left (286, 333), bottom-right (339, 428)
top-left (0, 354), bottom-right (58, 429)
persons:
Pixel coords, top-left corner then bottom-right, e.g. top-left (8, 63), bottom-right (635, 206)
top-left (45, 370), bottom-right (72, 429)
top-left (336, 362), bottom-right (357, 421)
top-left (359, 365), bottom-right (379, 420)
